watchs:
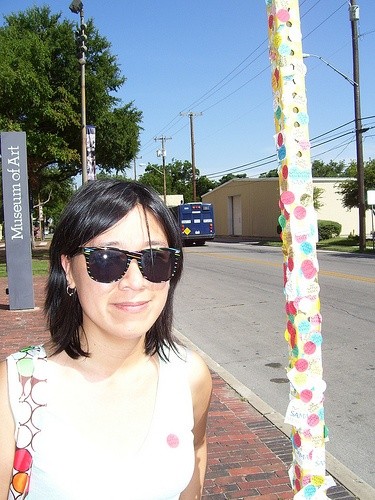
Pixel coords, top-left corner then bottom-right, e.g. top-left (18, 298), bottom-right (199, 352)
top-left (0, 175), bottom-right (215, 500)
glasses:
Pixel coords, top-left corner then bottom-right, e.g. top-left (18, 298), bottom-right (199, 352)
top-left (66, 246), bottom-right (181, 283)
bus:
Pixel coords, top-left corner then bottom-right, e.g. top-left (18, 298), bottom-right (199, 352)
top-left (167, 201), bottom-right (215, 247)
